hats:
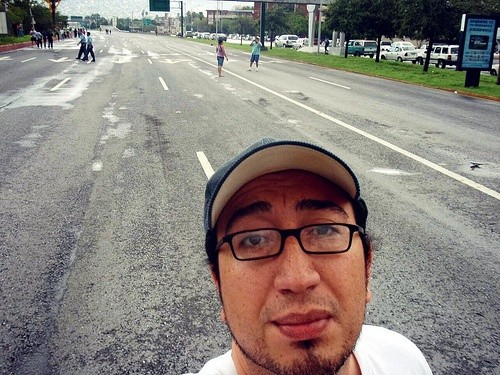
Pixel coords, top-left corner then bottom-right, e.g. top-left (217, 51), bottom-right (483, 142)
top-left (203, 135), bottom-right (369, 234)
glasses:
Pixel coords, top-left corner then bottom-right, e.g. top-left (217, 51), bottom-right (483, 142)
top-left (216, 222), bottom-right (365, 260)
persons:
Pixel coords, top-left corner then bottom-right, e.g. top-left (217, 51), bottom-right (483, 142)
top-left (195, 136), bottom-right (434, 375)
top-left (42, 31), bottom-right (47, 47)
top-left (81, 32), bottom-right (96, 62)
top-left (17, 22), bottom-right (24, 37)
top-left (246, 38), bottom-right (262, 72)
top-left (35, 30), bottom-right (42, 48)
top-left (215, 37), bottom-right (228, 77)
top-left (55, 25), bottom-right (111, 49)
top-left (75, 29), bottom-right (88, 60)
top-left (324, 37), bottom-right (329, 53)
top-left (30, 28), bottom-right (37, 48)
top-left (47, 30), bottom-right (54, 47)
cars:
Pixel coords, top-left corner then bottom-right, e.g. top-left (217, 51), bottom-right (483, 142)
top-left (164, 27), bottom-right (271, 42)
top-left (490, 63), bottom-right (499, 74)
top-left (380, 44), bottom-right (416, 63)
top-left (380, 39), bottom-right (417, 49)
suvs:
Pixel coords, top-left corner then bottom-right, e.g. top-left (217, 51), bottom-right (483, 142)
top-left (275, 34), bottom-right (300, 48)
top-left (344, 39), bottom-right (378, 58)
top-left (416, 42), bottom-right (460, 69)
